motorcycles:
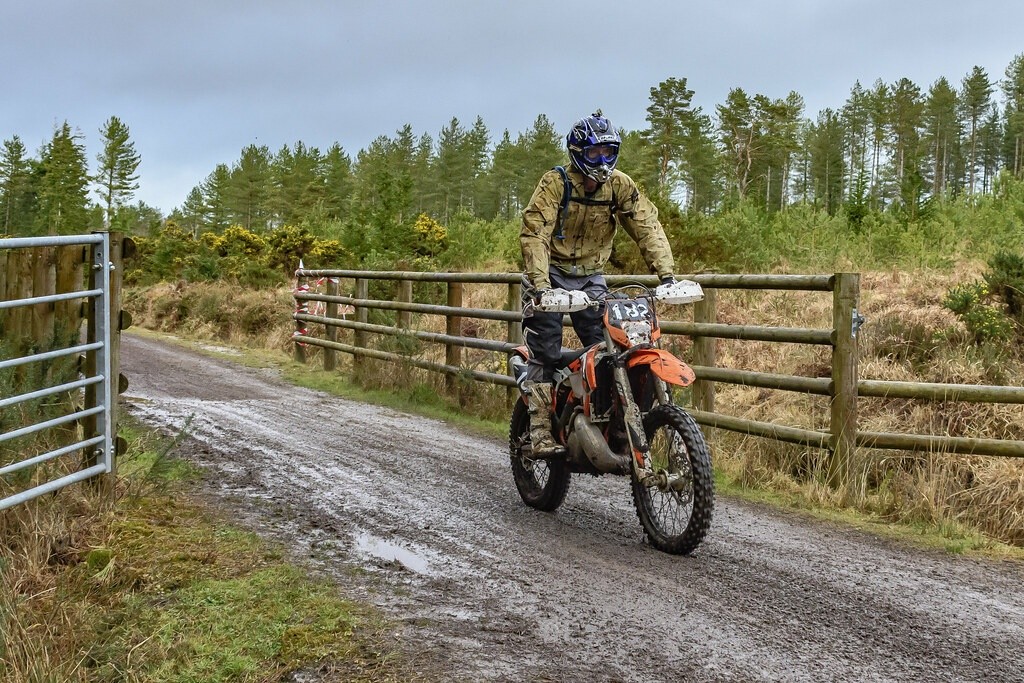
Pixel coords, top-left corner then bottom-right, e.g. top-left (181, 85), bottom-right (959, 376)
top-left (509, 281), bottom-right (716, 555)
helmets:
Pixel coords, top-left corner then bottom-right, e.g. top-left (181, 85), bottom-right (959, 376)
top-left (565, 108), bottom-right (622, 185)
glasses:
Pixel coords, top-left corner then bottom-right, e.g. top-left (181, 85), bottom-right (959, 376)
top-left (581, 144), bottom-right (619, 164)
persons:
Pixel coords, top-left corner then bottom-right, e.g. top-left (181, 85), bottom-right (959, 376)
top-left (518, 111), bottom-right (676, 454)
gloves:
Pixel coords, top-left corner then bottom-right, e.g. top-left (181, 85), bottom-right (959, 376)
top-left (536, 288), bottom-right (553, 303)
top-left (662, 277), bottom-right (678, 285)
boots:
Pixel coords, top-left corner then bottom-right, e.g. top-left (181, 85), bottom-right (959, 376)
top-left (527, 381), bottom-right (565, 456)
